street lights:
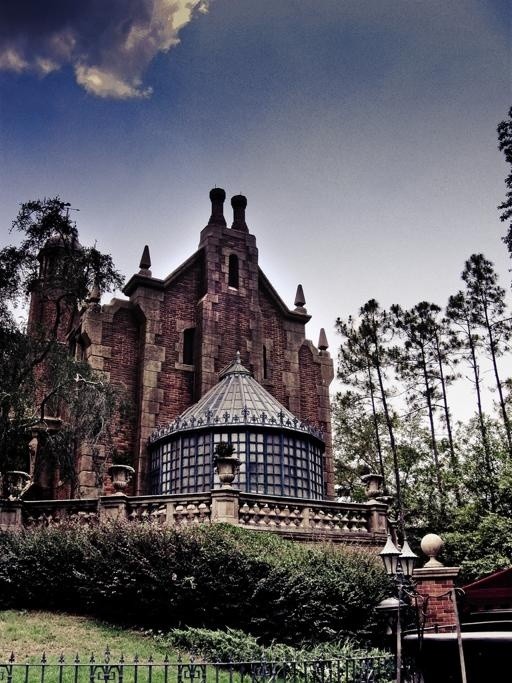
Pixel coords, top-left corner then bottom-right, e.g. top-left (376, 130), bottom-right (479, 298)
top-left (379, 534), bottom-right (418, 681)
